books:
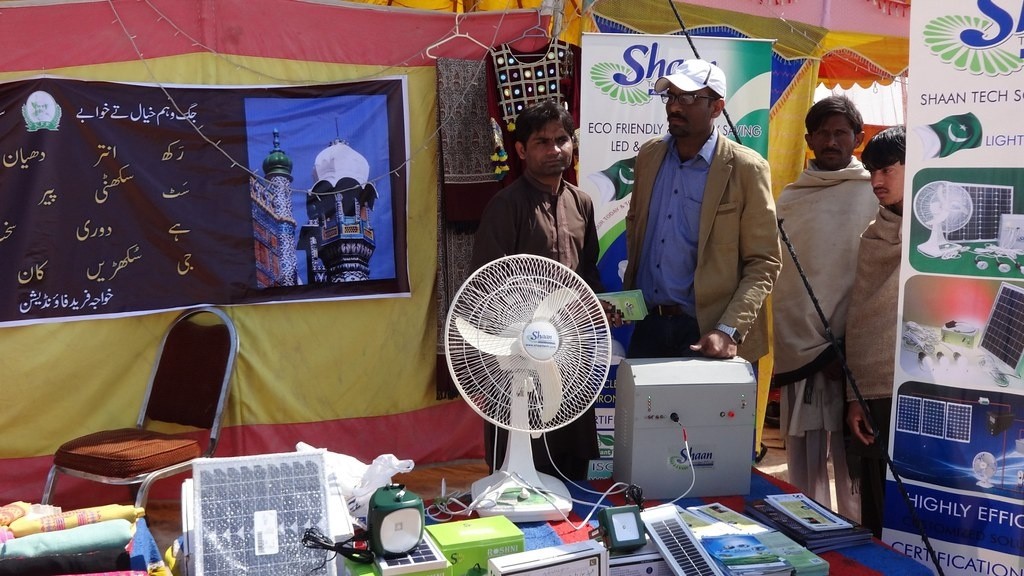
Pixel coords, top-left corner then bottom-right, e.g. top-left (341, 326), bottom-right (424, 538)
top-left (596, 290), bottom-right (648, 321)
top-left (676, 492), bottom-right (874, 576)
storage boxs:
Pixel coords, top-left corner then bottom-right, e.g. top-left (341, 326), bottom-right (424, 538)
top-left (343, 515), bottom-right (672, 576)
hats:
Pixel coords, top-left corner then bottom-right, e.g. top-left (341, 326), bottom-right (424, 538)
top-left (653, 59), bottom-right (727, 100)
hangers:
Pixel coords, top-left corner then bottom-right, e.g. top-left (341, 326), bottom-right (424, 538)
top-left (506, 8), bottom-right (553, 57)
top-left (426, 13), bottom-right (495, 63)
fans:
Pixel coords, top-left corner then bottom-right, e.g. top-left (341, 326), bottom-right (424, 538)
top-left (913, 180), bottom-right (973, 257)
top-left (441, 254), bottom-right (614, 526)
top-left (973, 451), bottom-right (997, 489)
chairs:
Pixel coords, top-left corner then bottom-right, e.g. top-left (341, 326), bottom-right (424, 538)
top-left (41, 307), bottom-right (240, 520)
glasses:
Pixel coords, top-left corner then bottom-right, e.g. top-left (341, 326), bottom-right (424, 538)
top-left (661, 93), bottom-right (717, 106)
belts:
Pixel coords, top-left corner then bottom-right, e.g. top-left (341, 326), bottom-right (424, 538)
top-left (646, 305), bottom-right (685, 317)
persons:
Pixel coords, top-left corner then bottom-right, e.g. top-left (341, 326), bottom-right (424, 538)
top-left (473, 99), bottom-right (631, 480)
top-left (770, 98), bottom-right (880, 520)
top-left (621, 60), bottom-right (783, 359)
top-left (841, 125), bottom-right (907, 540)
top-left (713, 506), bottom-right (725, 513)
top-left (807, 518), bottom-right (820, 524)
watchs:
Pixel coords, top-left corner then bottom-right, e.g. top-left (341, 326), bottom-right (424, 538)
top-left (714, 324), bottom-right (741, 344)
